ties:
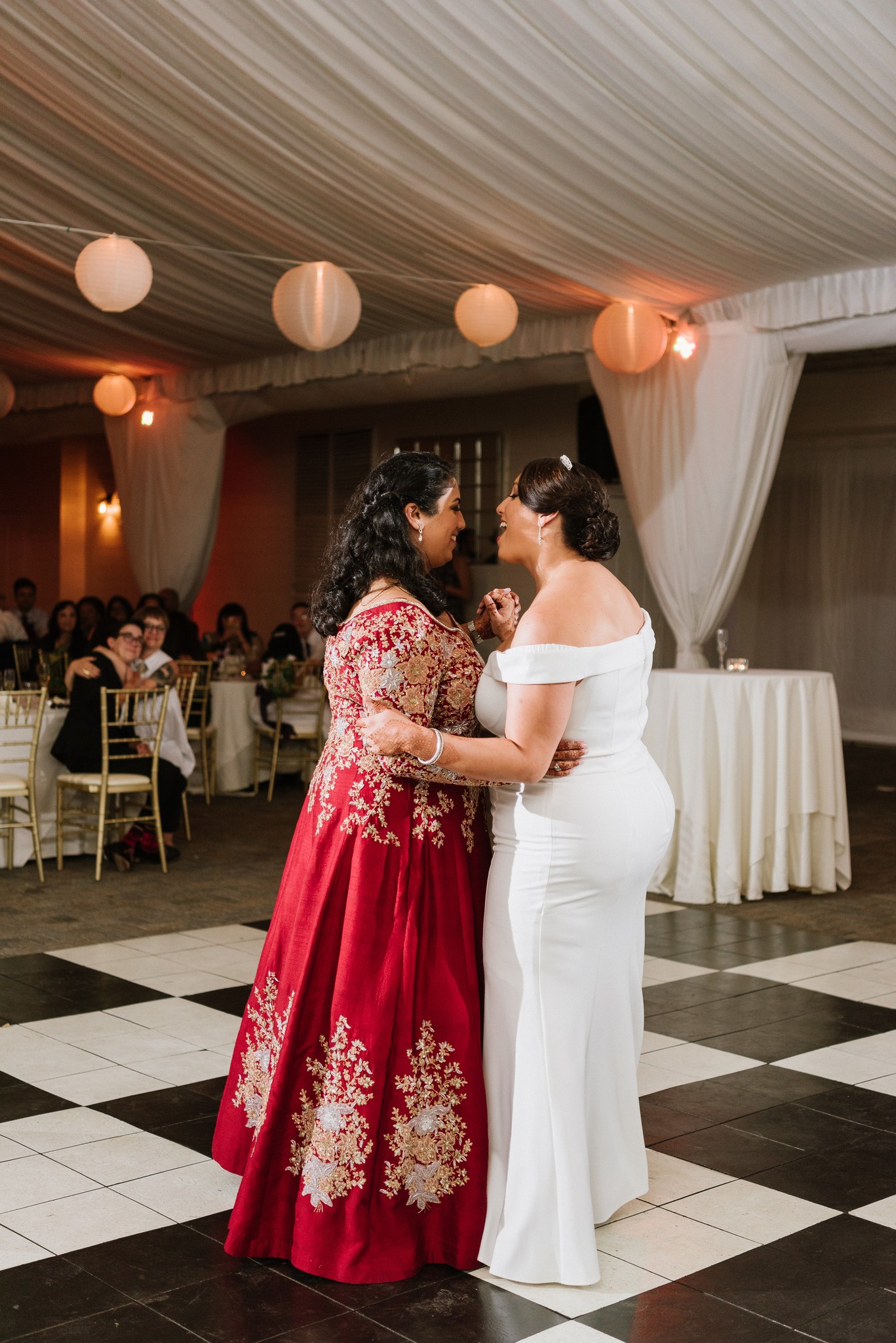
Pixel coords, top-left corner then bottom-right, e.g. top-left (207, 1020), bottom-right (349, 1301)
top-left (21, 613), bottom-right (27, 630)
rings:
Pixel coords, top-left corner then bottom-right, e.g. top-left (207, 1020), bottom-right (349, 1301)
top-left (82, 669), bottom-right (91, 675)
top-left (553, 761), bottom-right (562, 771)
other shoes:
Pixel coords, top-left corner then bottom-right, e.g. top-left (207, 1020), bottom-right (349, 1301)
top-left (104, 842), bottom-right (134, 871)
top-left (135, 841), bottom-right (180, 861)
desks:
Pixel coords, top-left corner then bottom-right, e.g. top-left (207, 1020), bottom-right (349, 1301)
top-left (209, 681), bottom-right (332, 785)
top-left (647, 669), bottom-right (852, 906)
top-left (0, 708), bottom-right (147, 868)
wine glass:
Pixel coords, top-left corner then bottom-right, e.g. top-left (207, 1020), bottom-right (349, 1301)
top-left (716, 627), bottom-right (728, 670)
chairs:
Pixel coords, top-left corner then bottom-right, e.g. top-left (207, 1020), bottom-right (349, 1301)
top-left (0, 659), bottom-right (326, 883)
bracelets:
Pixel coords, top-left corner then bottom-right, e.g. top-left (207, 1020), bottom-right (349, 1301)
top-left (418, 728), bottom-right (444, 765)
top-left (467, 620), bottom-right (484, 644)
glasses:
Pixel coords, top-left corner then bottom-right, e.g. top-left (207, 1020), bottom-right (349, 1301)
top-left (145, 623), bottom-right (167, 634)
top-left (119, 631), bottom-right (144, 645)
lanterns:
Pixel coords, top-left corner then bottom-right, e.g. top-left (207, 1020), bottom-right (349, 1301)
top-left (75, 238), bottom-right (153, 311)
top-left (455, 283), bottom-right (518, 347)
top-left (592, 302), bottom-right (667, 374)
top-left (272, 261), bottom-right (361, 351)
top-left (0, 373), bottom-right (14, 418)
top-left (93, 374), bottom-right (136, 416)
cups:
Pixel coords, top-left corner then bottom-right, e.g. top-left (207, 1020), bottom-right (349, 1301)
top-left (37, 663), bottom-right (50, 686)
top-left (4, 668), bottom-right (15, 691)
top-left (726, 656), bottom-right (748, 672)
top-left (21, 682), bottom-right (37, 711)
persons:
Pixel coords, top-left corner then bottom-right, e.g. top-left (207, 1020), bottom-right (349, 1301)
top-left (211, 451), bottom-right (520, 1284)
top-left (356, 454), bottom-right (676, 1286)
top-left (202, 601), bottom-right (263, 681)
top-left (0, 577), bottom-right (50, 641)
top-left (256, 621), bottom-right (306, 790)
top-left (107, 595), bottom-right (133, 637)
top-left (50, 617), bottom-right (188, 873)
top-left (132, 588), bottom-right (211, 727)
top-left (65, 606), bottom-right (197, 863)
top-left (38, 596), bottom-right (107, 696)
top-left (289, 602), bottom-right (328, 676)
